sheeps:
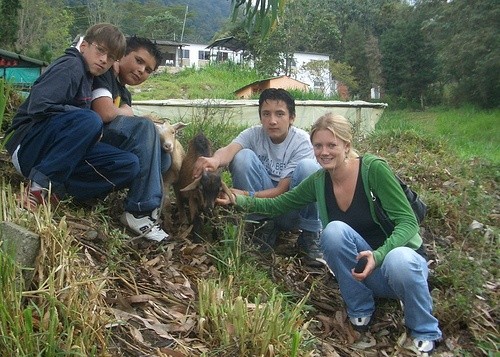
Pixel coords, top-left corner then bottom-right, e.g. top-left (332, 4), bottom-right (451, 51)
top-left (151, 121), bottom-right (189, 232)
top-left (180, 134), bottom-right (235, 246)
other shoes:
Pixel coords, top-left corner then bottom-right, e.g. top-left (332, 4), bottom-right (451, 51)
top-left (349, 311), bottom-right (374, 334)
top-left (14, 183), bottom-right (43, 213)
top-left (295, 230), bottom-right (324, 267)
top-left (250, 218), bottom-right (280, 261)
top-left (46, 192), bottom-right (60, 205)
top-left (411, 337), bottom-right (444, 356)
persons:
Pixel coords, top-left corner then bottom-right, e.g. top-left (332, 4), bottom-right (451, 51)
top-left (213, 112), bottom-right (442, 351)
top-left (90, 38), bottom-right (172, 241)
top-left (193, 88), bottom-right (327, 265)
top-left (4, 24), bottom-right (140, 214)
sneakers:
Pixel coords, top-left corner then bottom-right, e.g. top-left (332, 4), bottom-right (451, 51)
top-left (119, 211), bottom-right (169, 245)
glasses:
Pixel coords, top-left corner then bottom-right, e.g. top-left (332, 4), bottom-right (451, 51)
top-left (91, 42), bottom-right (117, 63)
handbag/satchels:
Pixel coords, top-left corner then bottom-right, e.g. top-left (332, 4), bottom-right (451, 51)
top-left (370, 174), bottom-right (427, 236)
